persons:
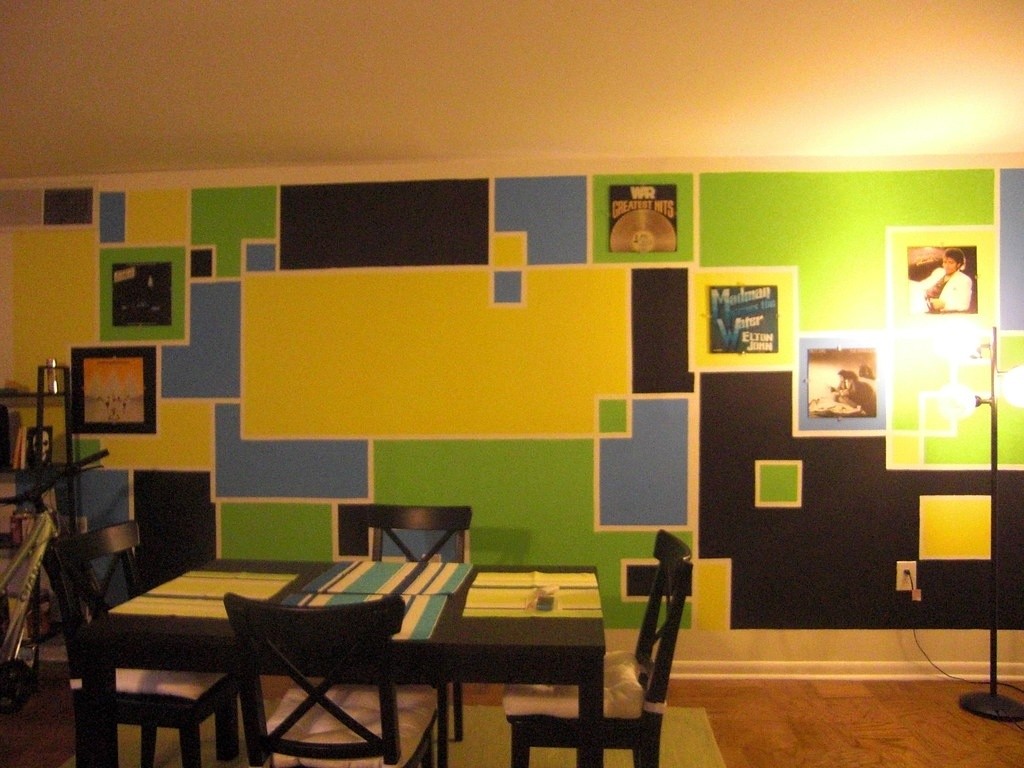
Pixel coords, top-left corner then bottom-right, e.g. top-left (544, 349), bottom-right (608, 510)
top-left (826, 369), bottom-right (876, 417)
top-left (914, 248), bottom-right (971, 313)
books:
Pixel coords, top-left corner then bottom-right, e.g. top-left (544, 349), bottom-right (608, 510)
top-left (13, 426), bottom-right (52, 470)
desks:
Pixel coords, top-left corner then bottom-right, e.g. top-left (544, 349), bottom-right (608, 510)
top-left (107, 559), bottom-right (607, 768)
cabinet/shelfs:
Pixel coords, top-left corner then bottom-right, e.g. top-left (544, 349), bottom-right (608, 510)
top-left (0, 363), bottom-right (81, 645)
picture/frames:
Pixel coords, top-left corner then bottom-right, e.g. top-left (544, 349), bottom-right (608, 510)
top-left (71, 344), bottom-right (158, 436)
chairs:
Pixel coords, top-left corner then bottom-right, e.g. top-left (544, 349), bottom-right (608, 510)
top-left (336, 504), bottom-right (474, 768)
top-left (45, 520), bottom-right (239, 768)
top-left (223, 592), bottom-right (439, 768)
top-left (502, 529), bottom-right (694, 768)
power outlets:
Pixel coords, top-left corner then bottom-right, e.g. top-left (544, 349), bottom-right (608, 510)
top-left (896, 561), bottom-right (917, 591)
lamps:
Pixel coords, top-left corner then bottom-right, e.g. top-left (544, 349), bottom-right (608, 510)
top-left (941, 325), bottom-right (1024, 723)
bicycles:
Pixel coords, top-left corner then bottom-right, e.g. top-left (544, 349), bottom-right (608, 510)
top-left (0, 448), bottom-right (111, 714)
